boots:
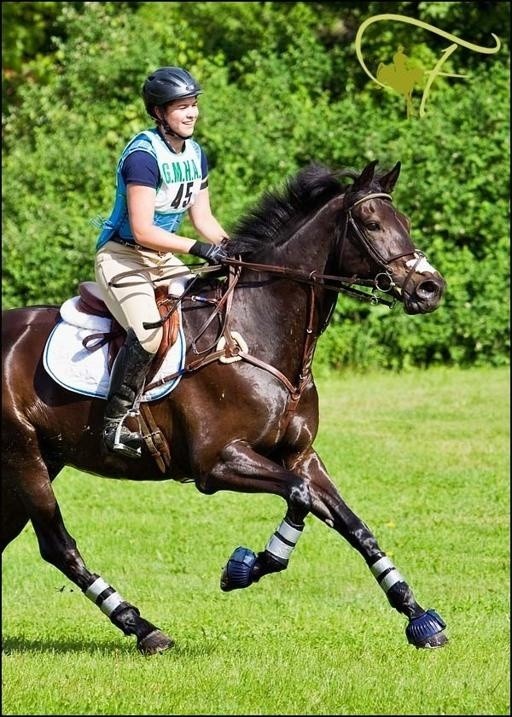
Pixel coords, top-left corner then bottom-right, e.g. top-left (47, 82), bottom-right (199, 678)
top-left (100, 328), bottom-right (155, 454)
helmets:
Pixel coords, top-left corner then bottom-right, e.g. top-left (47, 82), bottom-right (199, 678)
top-left (143, 68), bottom-right (204, 113)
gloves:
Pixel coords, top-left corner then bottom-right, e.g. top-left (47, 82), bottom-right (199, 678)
top-left (190, 239), bottom-right (229, 265)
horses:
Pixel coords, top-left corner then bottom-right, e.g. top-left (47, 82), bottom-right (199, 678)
top-left (0, 159), bottom-right (449, 657)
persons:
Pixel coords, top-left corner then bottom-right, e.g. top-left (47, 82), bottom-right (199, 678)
top-left (94, 66), bottom-right (232, 452)
